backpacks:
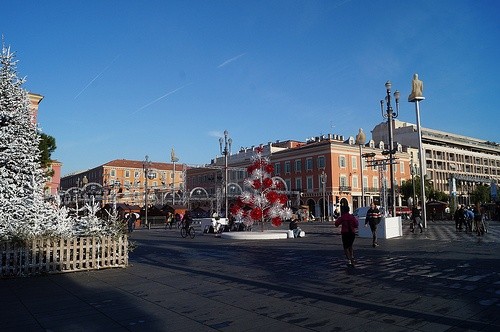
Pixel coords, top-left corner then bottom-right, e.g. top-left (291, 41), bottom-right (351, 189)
top-left (185, 214), bottom-right (192, 223)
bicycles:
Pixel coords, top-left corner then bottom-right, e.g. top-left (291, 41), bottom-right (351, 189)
top-left (180, 219), bottom-right (195, 240)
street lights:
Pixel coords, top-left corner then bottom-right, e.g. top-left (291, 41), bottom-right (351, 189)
top-left (320, 171), bottom-right (328, 220)
top-left (218, 130), bottom-right (232, 218)
top-left (142, 156), bottom-right (156, 227)
top-left (380, 80), bottom-right (400, 217)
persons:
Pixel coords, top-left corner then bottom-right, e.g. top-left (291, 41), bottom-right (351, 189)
top-left (334, 205), bottom-right (359, 265)
top-left (364, 203), bottom-right (382, 247)
top-left (289, 219), bottom-right (300, 238)
top-left (164, 210), bottom-right (183, 229)
top-left (409, 206), bottom-right (424, 233)
top-left (127, 211), bottom-right (136, 233)
top-left (432, 207), bottom-right (436, 222)
top-left (182, 211), bottom-right (192, 230)
top-left (472, 201), bottom-right (485, 236)
top-left (454, 205), bottom-right (477, 232)
top-left (228, 212), bottom-right (233, 231)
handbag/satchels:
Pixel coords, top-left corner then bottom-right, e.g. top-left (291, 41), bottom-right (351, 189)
top-left (410, 222), bottom-right (414, 230)
top-left (288, 229), bottom-right (294, 239)
top-left (298, 230), bottom-right (306, 238)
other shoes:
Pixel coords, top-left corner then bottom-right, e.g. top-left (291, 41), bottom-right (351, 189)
top-left (373, 244), bottom-right (379, 248)
top-left (347, 259), bottom-right (353, 264)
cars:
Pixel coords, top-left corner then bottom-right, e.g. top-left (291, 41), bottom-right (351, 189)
top-left (425, 201), bottom-right (449, 212)
top-left (352, 205), bottom-right (412, 217)
